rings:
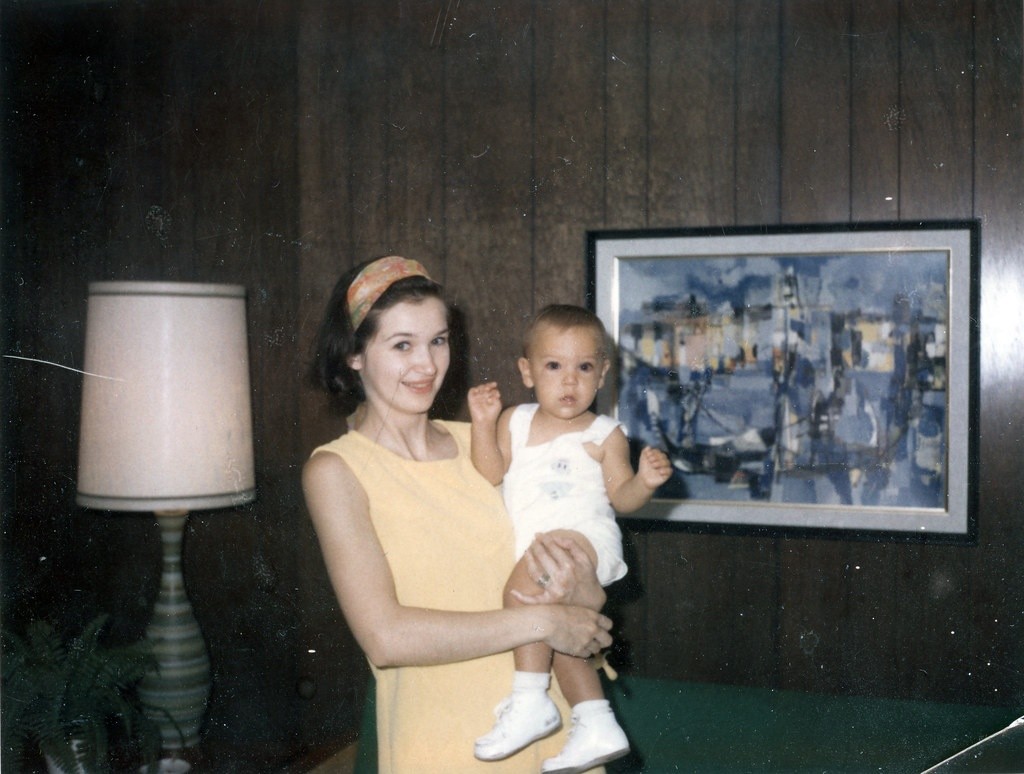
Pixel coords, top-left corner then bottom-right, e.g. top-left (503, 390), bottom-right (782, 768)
top-left (537, 574), bottom-right (549, 585)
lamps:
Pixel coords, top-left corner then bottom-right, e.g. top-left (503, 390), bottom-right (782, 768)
top-left (73, 279), bottom-right (262, 768)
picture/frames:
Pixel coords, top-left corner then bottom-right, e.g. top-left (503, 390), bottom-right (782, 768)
top-left (582, 218), bottom-right (983, 546)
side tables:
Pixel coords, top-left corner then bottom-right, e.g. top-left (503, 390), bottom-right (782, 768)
top-left (203, 714), bottom-right (359, 774)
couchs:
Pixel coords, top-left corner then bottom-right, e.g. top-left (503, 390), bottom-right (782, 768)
top-left (352, 672), bottom-right (1024, 774)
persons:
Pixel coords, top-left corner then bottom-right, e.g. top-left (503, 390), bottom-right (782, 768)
top-left (466, 304), bottom-right (673, 774)
top-left (301, 254), bottom-right (613, 774)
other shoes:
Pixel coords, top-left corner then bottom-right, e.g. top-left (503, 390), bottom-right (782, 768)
top-left (475, 697), bottom-right (561, 763)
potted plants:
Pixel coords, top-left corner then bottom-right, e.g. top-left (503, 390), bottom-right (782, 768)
top-left (0, 614), bottom-right (169, 774)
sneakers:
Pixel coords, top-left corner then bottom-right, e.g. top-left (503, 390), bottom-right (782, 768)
top-left (540, 708), bottom-right (631, 772)
